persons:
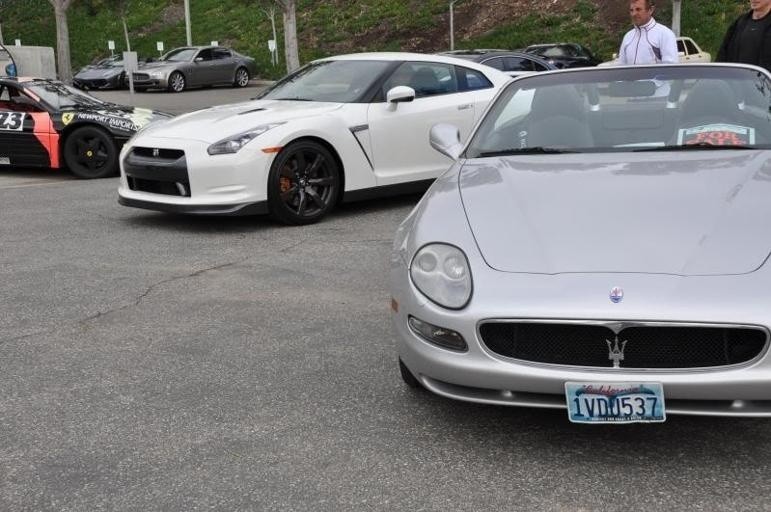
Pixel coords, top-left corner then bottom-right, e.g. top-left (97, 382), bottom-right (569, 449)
top-left (714, 0), bottom-right (771, 70)
top-left (615, 0), bottom-right (680, 66)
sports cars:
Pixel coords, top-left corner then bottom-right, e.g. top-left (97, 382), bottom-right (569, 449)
top-left (72, 54), bottom-right (157, 92)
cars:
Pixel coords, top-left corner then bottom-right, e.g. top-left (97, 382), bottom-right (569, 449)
top-left (434, 48), bottom-right (508, 60)
top-left (523, 42), bottom-right (601, 70)
top-left (471, 52), bottom-right (558, 76)
top-left (117, 50), bottom-right (537, 227)
top-left (0, 76), bottom-right (182, 180)
top-left (390, 62), bottom-right (771, 425)
top-left (123, 45), bottom-right (258, 94)
top-left (675, 36), bottom-right (712, 64)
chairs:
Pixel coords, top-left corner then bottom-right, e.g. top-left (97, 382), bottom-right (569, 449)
top-left (526, 78), bottom-right (742, 149)
top-left (410, 66), bottom-right (446, 97)
